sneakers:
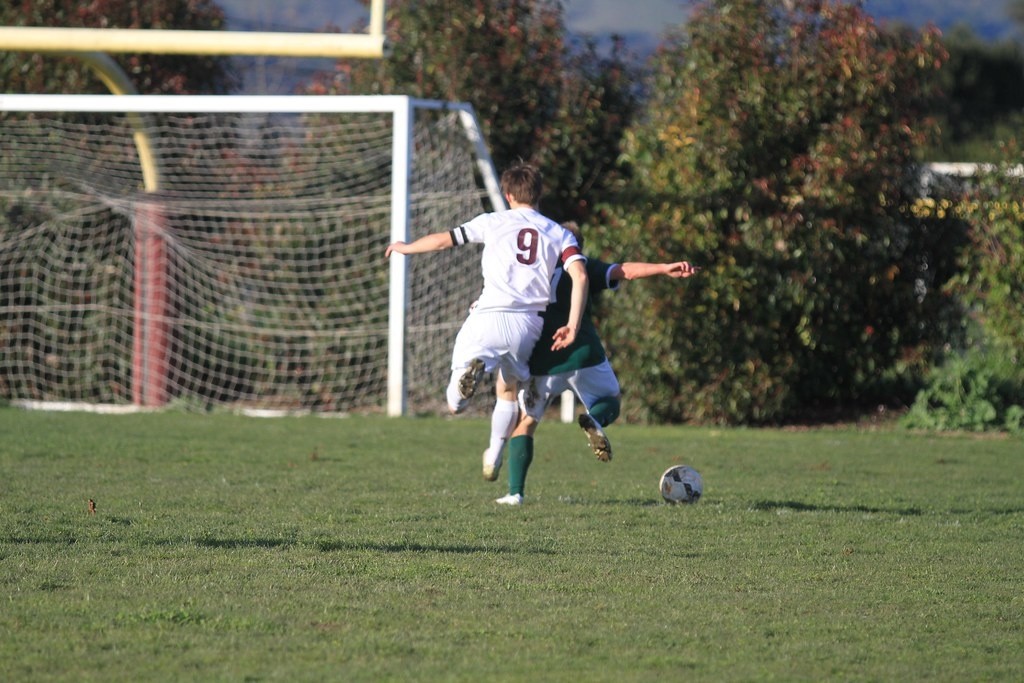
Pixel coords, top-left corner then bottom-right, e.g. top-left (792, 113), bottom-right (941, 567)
top-left (482, 448), bottom-right (502, 480)
top-left (578, 413), bottom-right (613, 462)
top-left (493, 493), bottom-right (524, 506)
top-left (457, 359), bottom-right (485, 399)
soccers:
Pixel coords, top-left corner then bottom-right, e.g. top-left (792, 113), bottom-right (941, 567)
top-left (659, 464), bottom-right (703, 506)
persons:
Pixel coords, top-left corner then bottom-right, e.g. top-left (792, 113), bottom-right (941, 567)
top-left (494, 222), bottom-right (703, 506)
top-left (385, 162), bottom-right (589, 482)
